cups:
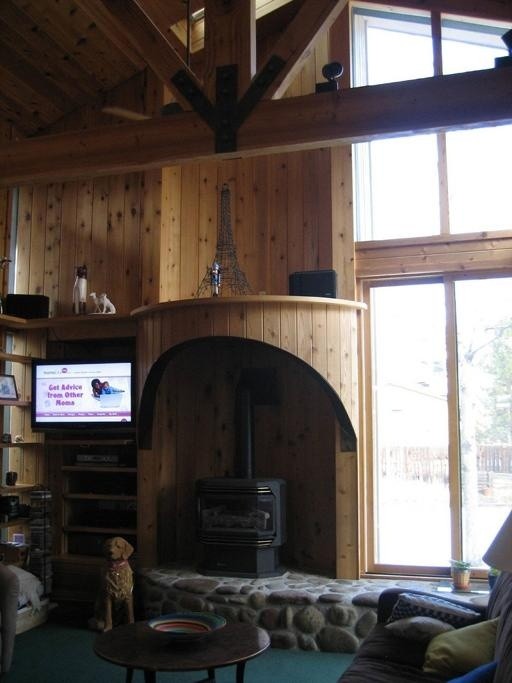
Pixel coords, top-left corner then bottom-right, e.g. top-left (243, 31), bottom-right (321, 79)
top-left (6, 471), bottom-right (18, 486)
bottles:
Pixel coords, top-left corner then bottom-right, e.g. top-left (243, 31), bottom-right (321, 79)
top-left (73, 267), bottom-right (88, 314)
top-left (210, 262), bottom-right (222, 297)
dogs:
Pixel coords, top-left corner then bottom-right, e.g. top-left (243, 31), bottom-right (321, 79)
top-left (88, 537), bottom-right (134, 632)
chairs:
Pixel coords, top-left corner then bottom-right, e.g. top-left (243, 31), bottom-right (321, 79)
top-left (1, 564), bottom-right (20, 674)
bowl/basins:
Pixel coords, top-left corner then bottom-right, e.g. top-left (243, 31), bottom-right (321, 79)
top-left (147, 612), bottom-right (228, 641)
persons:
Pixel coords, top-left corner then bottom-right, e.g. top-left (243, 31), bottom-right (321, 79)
top-left (100, 382), bottom-right (113, 394)
top-left (91, 379), bottom-right (124, 398)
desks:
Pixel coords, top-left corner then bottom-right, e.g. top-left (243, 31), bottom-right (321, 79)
top-left (93, 614), bottom-right (271, 683)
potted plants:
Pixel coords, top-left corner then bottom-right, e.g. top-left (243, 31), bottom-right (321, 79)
top-left (450, 559), bottom-right (472, 591)
top-left (488, 567), bottom-right (501, 587)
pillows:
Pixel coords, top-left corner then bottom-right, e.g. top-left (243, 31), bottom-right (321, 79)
top-left (383, 616), bottom-right (456, 645)
top-left (385, 592), bottom-right (481, 629)
top-left (422, 616), bottom-right (500, 678)
top-left (443, 659), bottom-right (498, 683)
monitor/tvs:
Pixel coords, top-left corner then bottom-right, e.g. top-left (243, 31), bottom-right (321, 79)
top-left (31, 359), bottom-right (133, 432)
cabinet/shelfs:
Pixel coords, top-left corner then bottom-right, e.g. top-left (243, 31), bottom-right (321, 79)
top-left (49, 435), bottom-right (176, 602)
top-left (0, 315), bottom-right (63, 635)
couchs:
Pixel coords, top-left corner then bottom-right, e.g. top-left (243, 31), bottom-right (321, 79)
top-left (333, 570), bottom-right (511, 683)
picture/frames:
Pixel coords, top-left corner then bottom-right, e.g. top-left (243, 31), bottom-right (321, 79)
top-left (0, 375), bottom-right (19, 401)
